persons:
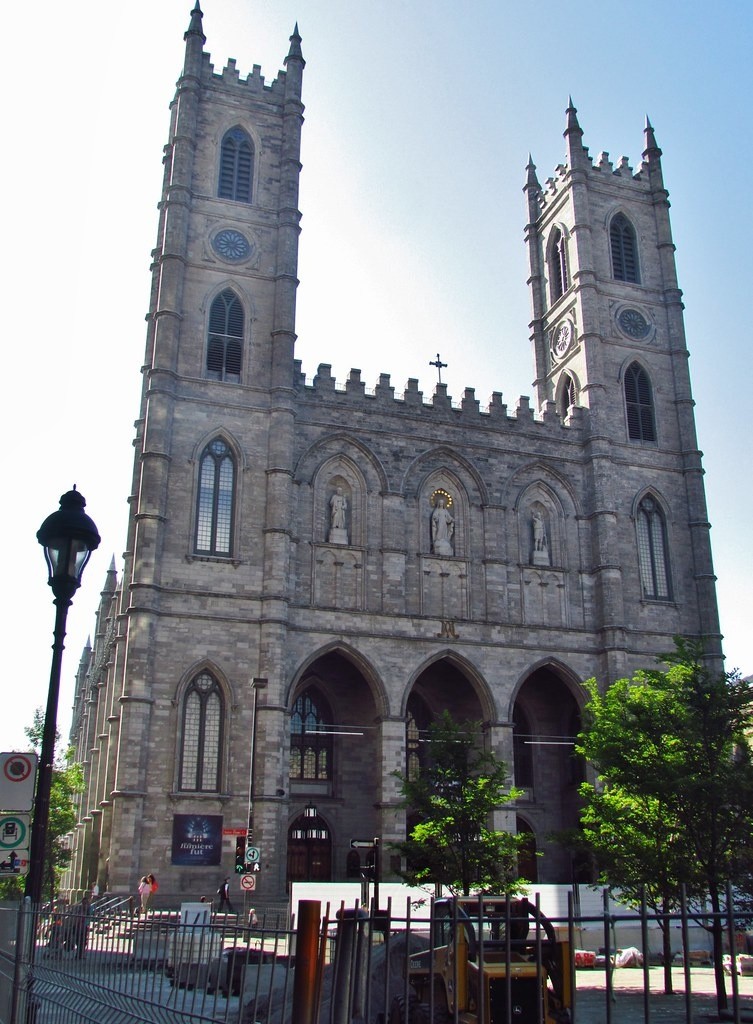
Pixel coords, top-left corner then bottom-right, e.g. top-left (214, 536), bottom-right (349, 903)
top-left (43, 881), bottom-right (100, 961)
top-left (248, 908), bottom-right (258, 929)
top-left (330, 486), bottom-right (348, 528)
top-left (531, 510), bottom-right (545, 550)
top-left (432, 500), bottom-right (455, 542)
top-left (216, 877), bottom-right (236, 914)
top-left (137, 873), bottom-right (158, 913)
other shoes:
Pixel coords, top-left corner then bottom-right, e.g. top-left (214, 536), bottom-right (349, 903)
top-left (142, 910), bottom-right (146, 913)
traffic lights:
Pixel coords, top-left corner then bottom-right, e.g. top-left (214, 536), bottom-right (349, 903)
top-left (247, 862), bottom-right (261, 872)
top-left (235, 836), bottom-right (245, 874)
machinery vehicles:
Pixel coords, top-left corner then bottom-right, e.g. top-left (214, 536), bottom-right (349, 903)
top-left (389, 890), bottom-right (584, 1024)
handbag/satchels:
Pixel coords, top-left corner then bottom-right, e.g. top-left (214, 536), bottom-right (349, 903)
top-left (139, 890), bottom-right (143, 894)
top-left (217, 889), bottom-right (221, 895)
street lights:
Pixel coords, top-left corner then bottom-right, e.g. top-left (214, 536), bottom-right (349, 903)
top-left (21, 482), bottom-right (102, 906)
top-left (241, 676), bottom-right (270, 942)
